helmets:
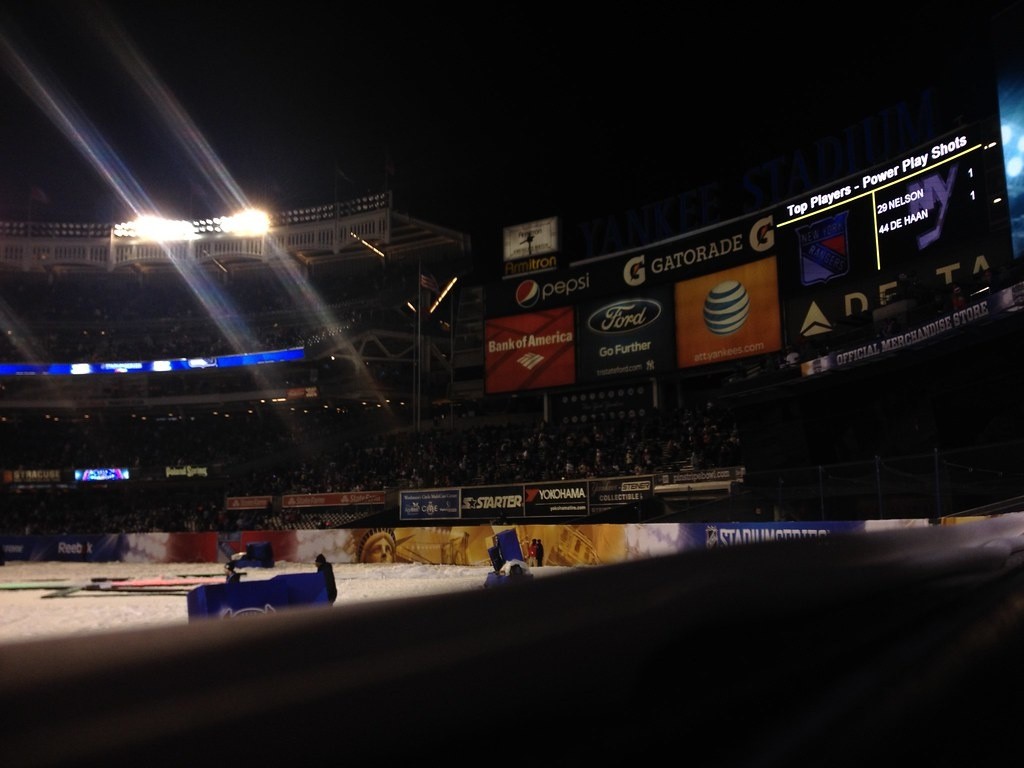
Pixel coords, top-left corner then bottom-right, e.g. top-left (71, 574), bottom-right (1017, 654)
top-left (509, 565), bottom-right (522, 576)
top-left (225, 562), bottom-right (235, 570)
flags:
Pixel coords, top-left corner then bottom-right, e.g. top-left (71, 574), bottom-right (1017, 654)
top-left (421, 270), bottom-right (438, 291)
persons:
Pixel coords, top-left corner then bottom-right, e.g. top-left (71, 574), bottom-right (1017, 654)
top-left (529, 539), bottom-right (543, 567)
top-left (0, 287), bottom-right (298, 364)
top-left (786, 347), bottom-right (800, 366)
top-left (950, 268), bottom-right (1000, 308)
top-left (881, 318), bottom-right (896, 336)
top-left (500, 559), bottom-right (529, 575)
top-left (0, 403), bottom-right (743, 534)
top-left (315, 554), bottom-right (337, 607)
top-left (224, 561), bottom-right (240, 583)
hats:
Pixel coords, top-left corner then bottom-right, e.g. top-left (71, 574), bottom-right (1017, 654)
top-left (315, 555), bottom-right (325, 562)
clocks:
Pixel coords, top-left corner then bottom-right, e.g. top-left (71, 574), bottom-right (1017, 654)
top-left (502, 216), bottom-right (563, 261)
